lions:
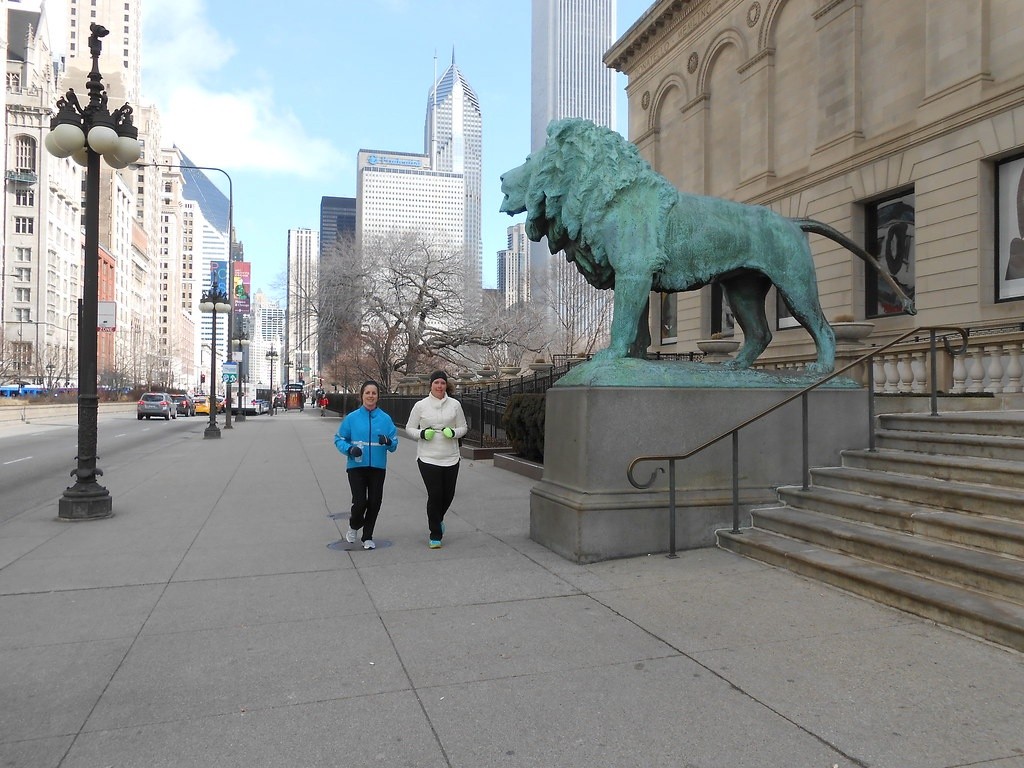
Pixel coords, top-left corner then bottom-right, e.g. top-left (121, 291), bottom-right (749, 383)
top-left (498, 119), bottom-right (917, 372)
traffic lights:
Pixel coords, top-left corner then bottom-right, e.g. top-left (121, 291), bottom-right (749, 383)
top-left (201, 374), bottom-right (206, 383)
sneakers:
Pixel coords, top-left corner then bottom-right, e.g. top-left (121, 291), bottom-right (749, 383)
top-left (346, 524), bottom-right (357, 544)
top-left (429, 540), bottom-right (442, 548)
top-left (362, 540), bottom-right (375, 549)
top-left (441, 521), bottom-right (446, 534)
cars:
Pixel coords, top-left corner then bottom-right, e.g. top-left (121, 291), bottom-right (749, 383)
top-left (203, 392), bottom-right (227, 414)
top-left (273, 389), bottom-right (286, 409)
top-left (192, 396), bottom-right (211, 416)
top-left (137, 392), bottom-right (178, 420)
top-left (168, 393), bottom-right (197, 417)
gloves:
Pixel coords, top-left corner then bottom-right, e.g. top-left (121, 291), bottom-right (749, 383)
top-left (421, 427), bottom-right (435, 441)
top-left (442, 427), bottom-right (455, 437)
top-left (349, 447), bottom-right (363, 457)
top-left (378, 435), bottom-right (392, 446)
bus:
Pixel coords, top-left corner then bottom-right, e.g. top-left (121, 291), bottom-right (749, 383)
top-left (0, 384), bottom-right (78, 398)
top-left (230, 383), bottom-right (275, 416)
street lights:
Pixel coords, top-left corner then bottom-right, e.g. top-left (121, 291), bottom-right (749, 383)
top-left (284, 360), bottom-right (291, 390)
top-left (296, 362), bottom-right (304, 383)
top-left (44, 22), bottom-right (143, 520)
top-left (264, 343), bottom-right (279, 416)
top-left (231, 331), bottom-right (251, 421)
top-left (65, 313), bottom-right (77, 388)
top-left (168, 350), bottom-right (181, 389)
top-left (198, 269), bottom-right (232, 440)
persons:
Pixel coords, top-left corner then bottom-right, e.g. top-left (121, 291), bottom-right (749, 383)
top-left (319, 394), bottom-right (328, 417)
top-left (268, 391), bottom-right (277, 416)
top-left (334, 381), bottom-right (399, 549)
top-left (311, 396), bottom-right (316, 408)
top-left (446, 381), bottom-right (463, 446)
top-left (406, 370), bottom-right (468, 548)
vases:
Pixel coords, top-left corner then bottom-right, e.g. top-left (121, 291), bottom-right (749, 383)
top-left (458, 373), bottom-right (475, 382)
top-left (499, 367), bottom-right (522, 380)
top-left (829, 322), bottom-right (876, 346)
top-left (567, 358), bottom-right (587, 368)
top-left (695, 341), bottom-right (741, 358)
top-left (477, 371), bottom-right (497, 382)
top-left (529, 363), bottom-right (554, 377)
